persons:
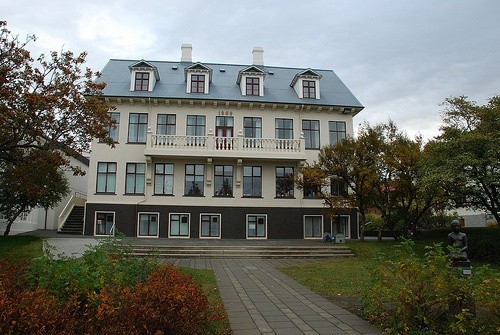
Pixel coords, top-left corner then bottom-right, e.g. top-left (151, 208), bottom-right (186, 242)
top-left (447, 220), bottom-right (468, 261)
